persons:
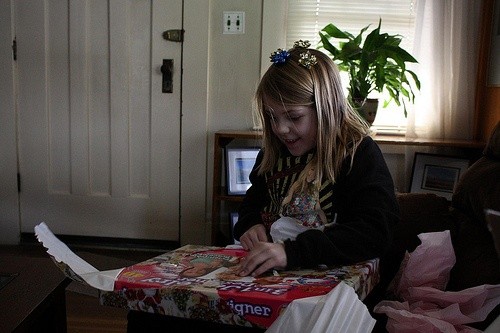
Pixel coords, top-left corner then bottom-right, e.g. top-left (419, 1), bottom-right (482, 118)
top-left (127, 39), bottom-right (406, 332)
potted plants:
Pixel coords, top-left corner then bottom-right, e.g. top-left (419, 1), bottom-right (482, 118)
top-left (316, 17), bottom-right (421, 126)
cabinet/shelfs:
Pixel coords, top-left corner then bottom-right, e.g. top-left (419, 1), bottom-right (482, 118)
top-left (211, 129), bottom-right (486, 246)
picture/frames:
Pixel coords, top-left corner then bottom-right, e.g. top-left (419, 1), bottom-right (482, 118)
top-left (409, 150), bottom-right (473, 202)
top-left (485, 0), bottom-right (500, 88)
top-left (228, 212), bottom-right (241, 245)
top-left (224, 146), bottom-right (263, 197)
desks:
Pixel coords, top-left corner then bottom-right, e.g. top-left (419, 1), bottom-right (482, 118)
top-left (0, 256), bottom-right (72, 333)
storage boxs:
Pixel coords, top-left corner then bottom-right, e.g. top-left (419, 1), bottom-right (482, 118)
top-left (35, 221), bottom-right (382, 333)
